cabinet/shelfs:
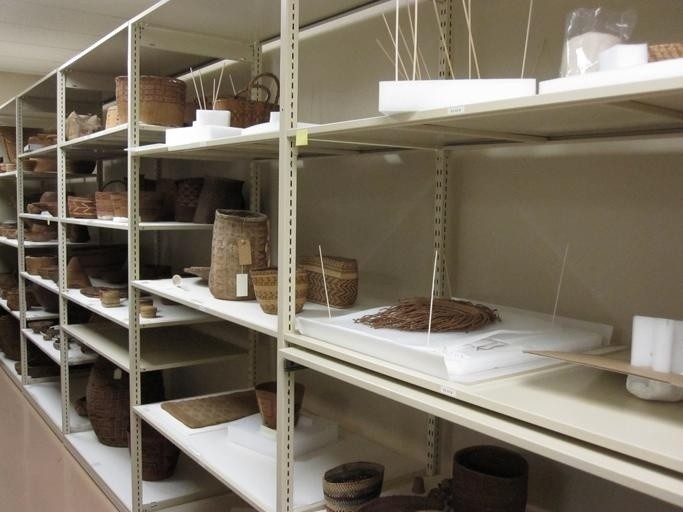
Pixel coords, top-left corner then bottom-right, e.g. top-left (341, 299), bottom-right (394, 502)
top-left (0, 1), bottom-right (682, 512)
